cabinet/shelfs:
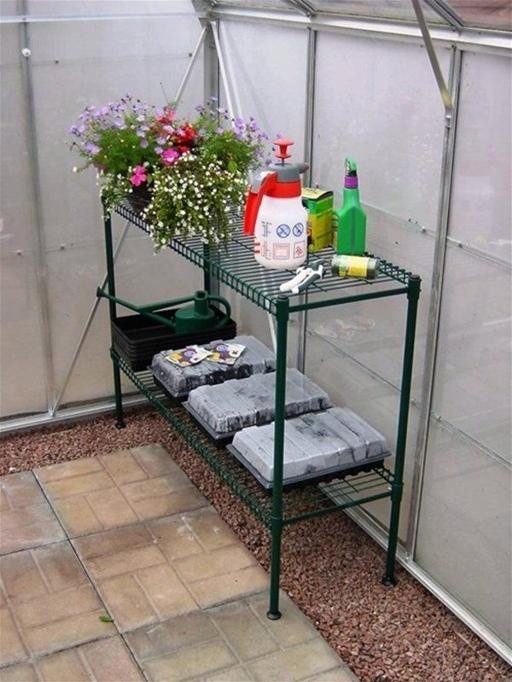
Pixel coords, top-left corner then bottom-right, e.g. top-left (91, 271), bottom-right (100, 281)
top-left (100, 181), bottom-right (421, 621)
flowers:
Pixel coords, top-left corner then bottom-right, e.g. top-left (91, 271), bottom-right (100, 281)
top-left (63, 94), bottom-right (282, 256)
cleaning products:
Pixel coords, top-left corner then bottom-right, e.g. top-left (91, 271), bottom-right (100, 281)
top-left (332, 158), bottom-right (367, 255)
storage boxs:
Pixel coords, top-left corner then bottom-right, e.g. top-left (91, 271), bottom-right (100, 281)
top-left (147, 335), bottom-right (277, 405)
top-left (181, 369), bottom-right (332, 449)
top-left (111, 303), bottom-right (237, 373)
top-left (226, 406), bottom-right (391, 496)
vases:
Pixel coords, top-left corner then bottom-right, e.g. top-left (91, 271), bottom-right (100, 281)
top-left (125, 189), bottom-right (156, 215)
